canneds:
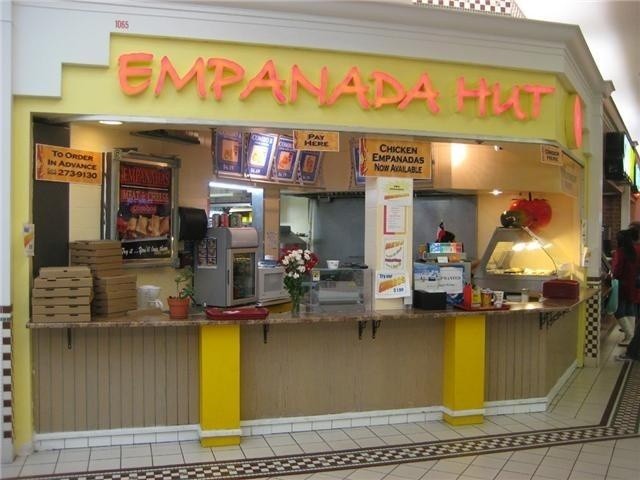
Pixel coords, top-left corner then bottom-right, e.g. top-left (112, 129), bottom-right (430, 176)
top-left (212, 214), bottom-right (220, 227)
top-left (229, 214), bottom-right (236, 227)
top-left (236, 214), bottom-right (242, 227)
top-left (220, 214), bottom-right (229, 227)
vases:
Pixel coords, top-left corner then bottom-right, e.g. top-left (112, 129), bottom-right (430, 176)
top-left (289, 287), bottom-right (301, 313)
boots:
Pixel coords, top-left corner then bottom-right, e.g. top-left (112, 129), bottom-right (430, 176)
top-left (617, 316), bottom-right (634, 346)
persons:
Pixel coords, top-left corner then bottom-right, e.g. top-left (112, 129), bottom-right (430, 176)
top-left (440, 231), bottom-right (482, 272)
top-left (603, 221), bottom-right (640, 362)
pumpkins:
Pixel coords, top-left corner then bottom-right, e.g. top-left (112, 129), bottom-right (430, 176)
top-left (510, 192), bottom-right (551, 228)
top-left (500, 211), bottom-right (523, 228)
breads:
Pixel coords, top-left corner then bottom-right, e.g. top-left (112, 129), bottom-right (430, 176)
top-left (116, 215), bottom-right (172, 240)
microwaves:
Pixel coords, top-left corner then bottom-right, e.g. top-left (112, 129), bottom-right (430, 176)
top-left (259, 264), bottom-right (292, 303)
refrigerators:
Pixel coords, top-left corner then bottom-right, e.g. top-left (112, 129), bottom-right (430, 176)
top-left (193, 227), bottom-right (258, 308)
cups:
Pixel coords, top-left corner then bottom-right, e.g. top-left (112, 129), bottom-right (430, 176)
top-left (481, 288), bottom-right (504, 309)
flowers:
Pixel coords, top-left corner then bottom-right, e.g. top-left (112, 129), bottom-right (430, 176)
top-left (279, 243), bottom-right (319, 313)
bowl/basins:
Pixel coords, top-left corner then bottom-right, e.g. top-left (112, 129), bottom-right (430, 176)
top-left (326, 260), bottom-right (340, 269)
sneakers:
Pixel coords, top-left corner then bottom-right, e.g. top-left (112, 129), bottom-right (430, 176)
top-left (614, 353), bottom-right (637, 361)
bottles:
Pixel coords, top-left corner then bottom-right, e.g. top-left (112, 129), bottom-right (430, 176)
top-left (521, 288), bottom-right (529, 305)
top-left (464, 283), bottom-right (472, 309)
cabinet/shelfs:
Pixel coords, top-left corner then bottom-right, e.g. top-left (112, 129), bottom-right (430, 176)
top-left (473, 227), bottom-right (571, 296)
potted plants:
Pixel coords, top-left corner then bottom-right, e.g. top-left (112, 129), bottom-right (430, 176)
top-left (168, 266), bottom-right (194, 319)
